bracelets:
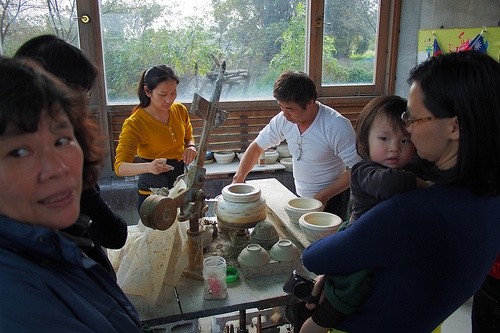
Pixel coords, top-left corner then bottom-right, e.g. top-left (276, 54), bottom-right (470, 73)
top-left (186, 144), bottom-right (196, 147)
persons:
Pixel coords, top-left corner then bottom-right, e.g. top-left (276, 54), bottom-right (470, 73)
top-left (300, 95), bottom-right (434, 333)
top-left (472, 255), bottom-right (500, 333)
top-left (13, 35), bottom-right (128, 284)
top-left (231, 71), bottom-right (363, 224)
top-left (301, 49), bottom-right (500, 333)
top-left (114, 63), bottom-right (198, 211)
top-left (0, 54), bottom-right (146, 333)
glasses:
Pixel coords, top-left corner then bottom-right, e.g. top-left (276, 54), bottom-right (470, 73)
top-left (401, 110), bottom-right (436, 126)
top-left (81, 88), bottom-right (93, 97)
top-left (297, 135), bottom-right (303, 161)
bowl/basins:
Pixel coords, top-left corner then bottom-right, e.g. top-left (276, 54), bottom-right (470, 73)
top-left (269, 239), bottom-right (301, 262)
top-left (213, 144), bottom-right (293, 172)
top-left (298, 212), bottom-right (343, 242)
top-left (283, 197), bottom-right (323, 224)
top-left (237, 244), bottom-right (270, 267)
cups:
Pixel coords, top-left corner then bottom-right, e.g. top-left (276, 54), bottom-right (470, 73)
top-left (257, 152), bottom-right (265, 167)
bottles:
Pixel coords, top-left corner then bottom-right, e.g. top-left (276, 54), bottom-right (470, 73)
top-left (202, 256), bottom-right (228, 300)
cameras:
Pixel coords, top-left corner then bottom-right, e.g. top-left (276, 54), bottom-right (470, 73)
top-left (282, 268), bottom-right (322, 304)
